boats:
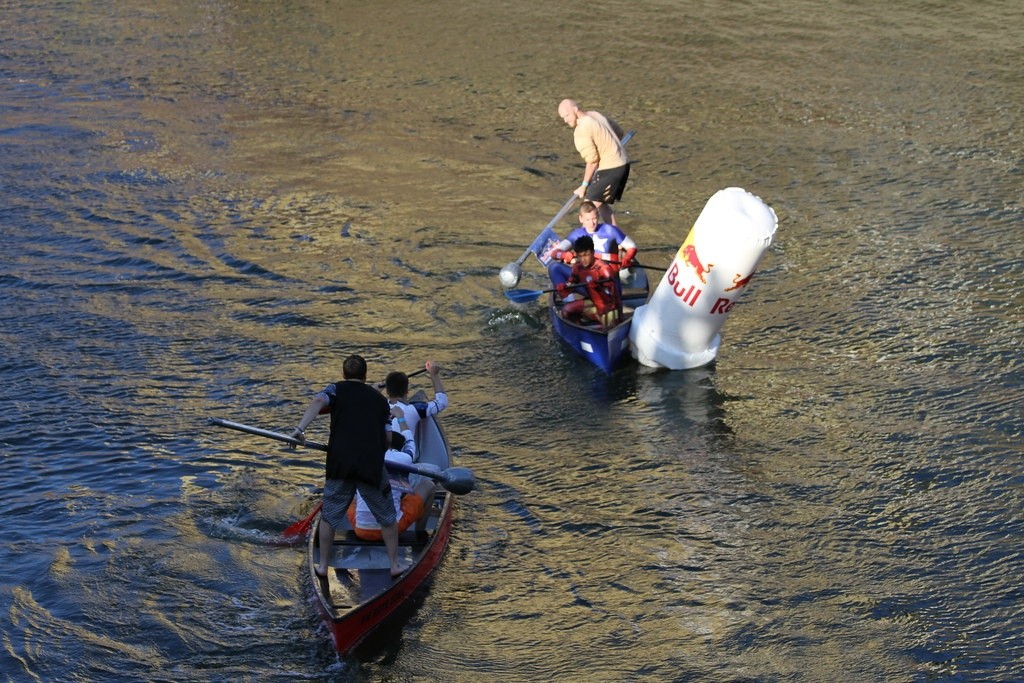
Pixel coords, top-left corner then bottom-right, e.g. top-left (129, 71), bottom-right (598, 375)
top-left (308, 390), bottom-right (453, 661)
top-left (549, 253), bottom-right (651, 377)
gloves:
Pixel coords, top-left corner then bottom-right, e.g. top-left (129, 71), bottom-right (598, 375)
top-left (551, 249), bottom-right (574, 263)
top-left (557, 283), bottom-right (569, 298)
top-left (621, 248), bottom-right (636, 267)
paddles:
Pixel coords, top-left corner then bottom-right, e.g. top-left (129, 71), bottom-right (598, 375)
top-left (504, 276), bottom-right (614, 305)
top-left (281, 500), bottom-right (324, 537)
top-left (318, 369), bottom-right (427, 416)
top-left (573, 255), bottom-right (669, 272)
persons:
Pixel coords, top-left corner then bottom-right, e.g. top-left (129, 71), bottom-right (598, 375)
top-left (285, 354), bottom-right (410, 577)
top-left (558, 98), bottom-right (631, 226)
top-left (548, 201), bottom-right (638, 299)
top-left (556, 234), bottom-right (618, 323)
top-left (372, 360), bottom-right (446, 461)
top-left (346, 406), bottom-right (435, 547)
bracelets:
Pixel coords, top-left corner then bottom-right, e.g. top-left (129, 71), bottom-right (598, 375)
top-left (297, 427), bottom-right (305, 434)
top-left (398, 417), bottom-right (406, 423)
top-left (582, 181), bottom-right (589, 186)
